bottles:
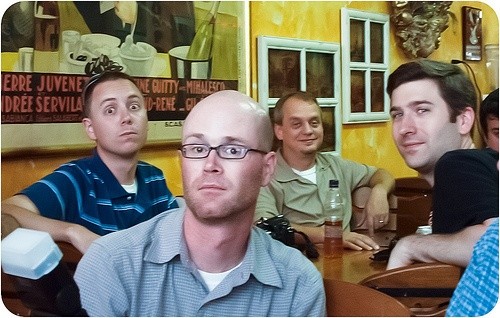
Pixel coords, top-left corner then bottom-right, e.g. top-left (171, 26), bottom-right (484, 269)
top-left (323, 180), bottom-right (344, 259)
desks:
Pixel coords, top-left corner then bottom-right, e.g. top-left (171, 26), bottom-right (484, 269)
top-left (311, 227), bottom-right (396, 283)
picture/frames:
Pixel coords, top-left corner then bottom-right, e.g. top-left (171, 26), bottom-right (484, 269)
top-left (462, 6), bottom-right (483, 61)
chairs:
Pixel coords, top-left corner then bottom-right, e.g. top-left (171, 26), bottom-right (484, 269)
top-left (352, 175), bottom-right (434, 246)
top-left (323, 279), bottom-right (415, 317)
top-left (357, 262), bottom-right (464, 317)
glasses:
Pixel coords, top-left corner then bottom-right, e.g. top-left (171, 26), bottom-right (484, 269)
top-left (369, 248), bottom-right (391, 262)
top-left (82, 73), bottom-right (104, 106)
top-left (177, 143), bottom-right (268, 159)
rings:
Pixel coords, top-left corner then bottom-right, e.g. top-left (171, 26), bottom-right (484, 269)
top-left (379, 220), bottom-right (384, 223)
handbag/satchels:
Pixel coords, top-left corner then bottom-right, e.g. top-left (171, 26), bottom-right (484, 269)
top-left (254, 214), bottom-right (320, 259)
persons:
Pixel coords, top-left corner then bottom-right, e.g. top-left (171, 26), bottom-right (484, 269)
top-left (73, 90), bottom-right (325, 317)
top-left (253, 93), bottom-right (394, 251)
top-left (480, 88), bottom-right (499, 150)
top-left (446, 220), bottom-right (499, 317)
top-left (384, 59), bottom-right (499, 271)
top-left (1, 71), bottom-right (180, 255)
